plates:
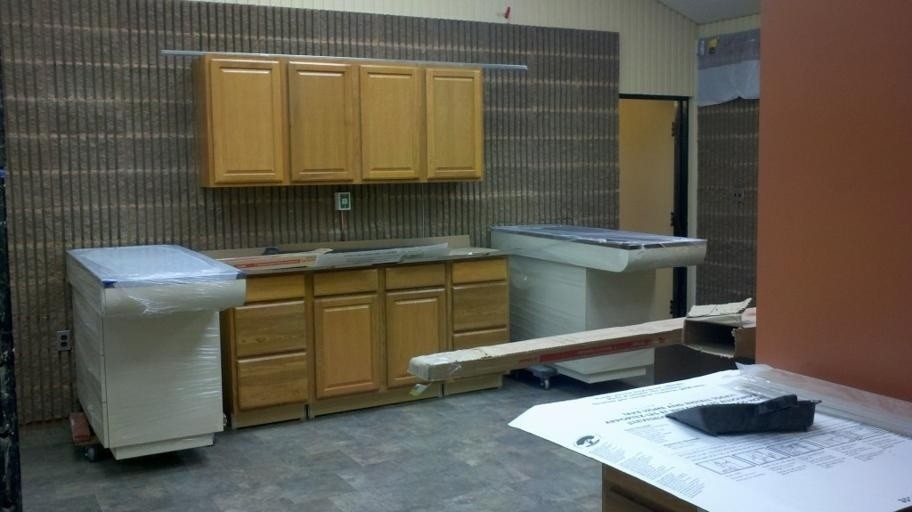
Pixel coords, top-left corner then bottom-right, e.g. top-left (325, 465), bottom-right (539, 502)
top-left (200, 56), bottom-right (484, 186)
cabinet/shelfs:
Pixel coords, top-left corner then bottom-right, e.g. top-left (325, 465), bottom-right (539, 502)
top-left (200, 56), bottom-right (484, 186)
top-left (215, 246), bottom-right (510, 431)
